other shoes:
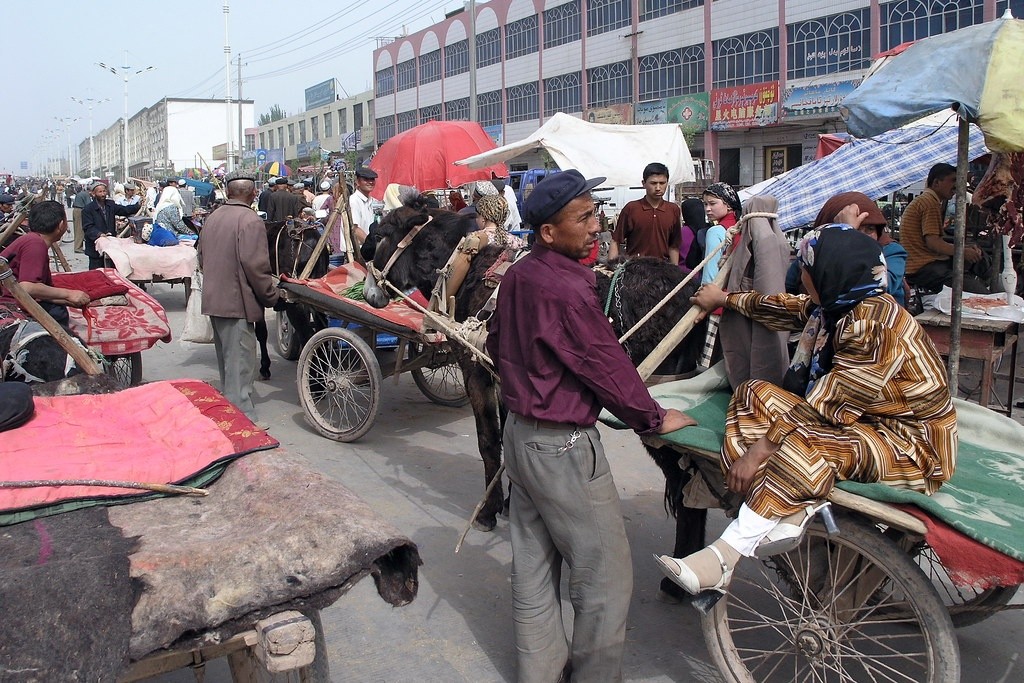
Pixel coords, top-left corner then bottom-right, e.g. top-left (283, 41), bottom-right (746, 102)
top-left (75, 248), bottom-right (85, 253)
top-left (254, 420), bottom-right (270, 432)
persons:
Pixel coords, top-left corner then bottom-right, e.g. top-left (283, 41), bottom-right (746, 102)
top-left (608, 163), bottom-right (743, 367)
top-left (436, 172), bottom-right (522, 323)
top-left (780, 190), bottom-right (907, 395)
top-left (900, 163), bottom-right (990, 295)
top-left (492, 169), bottom-right (698, 683)
top-left (0, 169), bottom-right (384, 270)
top-left (653, 222), bottom-right (957, 612)
top-left (0, 199), bottom-right (91, 432)
top-left (198, 170), bottom-right (285, 423)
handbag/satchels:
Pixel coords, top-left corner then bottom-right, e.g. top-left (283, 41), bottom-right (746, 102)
top-left (902, 280), bottom-right (924, 315)
top-left (181, 267), bottom-right (215, 343)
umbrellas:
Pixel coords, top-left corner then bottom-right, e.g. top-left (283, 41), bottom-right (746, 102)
top-left (366, 119), bottom-right (510, 200)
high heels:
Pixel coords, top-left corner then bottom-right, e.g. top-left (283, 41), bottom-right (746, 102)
top-left (755, 497), bottom-right (840, 556)
top-left (652, 545), bottom-right (730, 616)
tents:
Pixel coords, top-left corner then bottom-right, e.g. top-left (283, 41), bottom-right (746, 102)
top-left (837, 19), bottom-right (1024, 400)
top-left (737, 108), bottom-right (990, 232)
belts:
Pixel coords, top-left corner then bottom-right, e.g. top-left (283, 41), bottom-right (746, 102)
top-left (516, 415), bottom-right (585, 429)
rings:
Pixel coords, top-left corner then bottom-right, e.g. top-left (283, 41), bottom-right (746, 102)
top-left (698, 286), bottom-right (703, 291)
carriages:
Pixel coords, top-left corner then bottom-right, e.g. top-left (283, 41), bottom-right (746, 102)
top-left (0, 191), bottom-right (142, 398)
top-left (181, 202), bottom-right (474, 445)
top-left (360, 189), bottom-right (1024, 683)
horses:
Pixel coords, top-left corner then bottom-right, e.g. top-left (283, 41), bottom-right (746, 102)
top-left (182, 211), bottom-right (338, 407)
top-left (363, 184), bottom-right (724, 605)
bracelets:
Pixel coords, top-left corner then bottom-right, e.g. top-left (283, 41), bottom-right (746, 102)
top-left (724, 292), bottom-right (729, 309)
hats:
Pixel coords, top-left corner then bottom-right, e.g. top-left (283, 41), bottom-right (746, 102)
top-left (91, 182), bottom-right (107, 191)
top-left (225, 170), bottom-right (256, 183)
top-left (357, 168), bottom-right (378, 178)
top-left (158, 181), bottom-right (167, 187)
top-left (275, 178), bottom-right (288, 185)
top-left (124, 184), bottom-right (134, 190)
top-left (268, 176), bottom-right (282, 186)
top-left (168, 178), bottom-right (176, 183)
top-left (0, 194), bottom-right (16, 205)
top-left (321, 181), bottom-right (331, 191)
top-left (524, 169), bottom-right (606, 226)
top-left (294, 183), bottom-right (304, 189)
top-left (474, 180), bottom-right (500, 199)
top-left (302, 181), bottom-right (310, 188)
